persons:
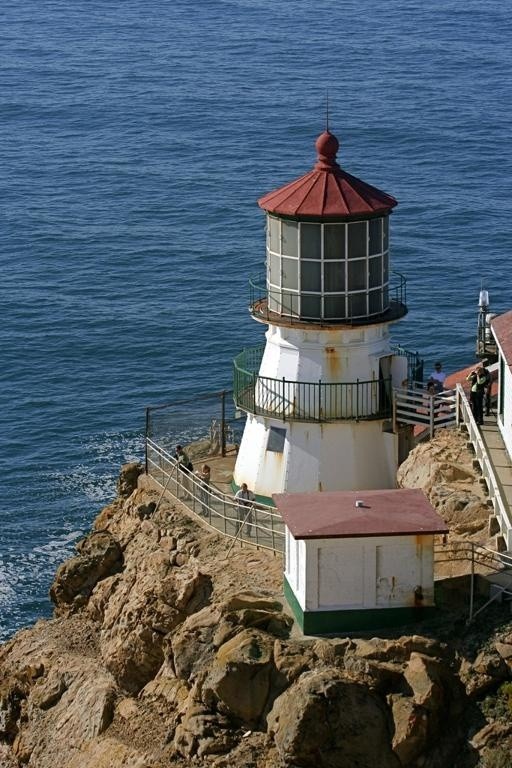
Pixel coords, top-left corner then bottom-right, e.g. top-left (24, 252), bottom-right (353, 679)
top-left (233, 481), bottom-right (256, 537)
top-left (193, 462), bottom-right (211, 518)
top-left (172, 444), bottom-right (194, 502)
top-left (465, 366), bottom-right (488, 426)
top-left (483, 369), bottom-right (493, 416)
top-left (426, 361), bottom-right (447, 419)
